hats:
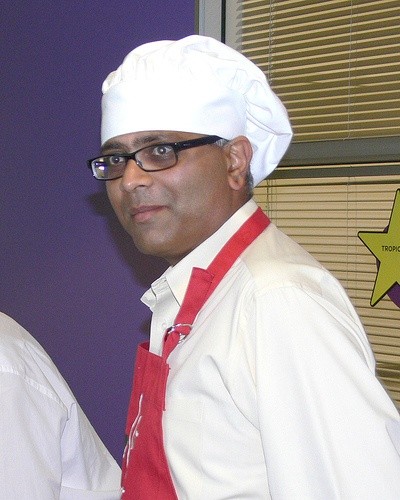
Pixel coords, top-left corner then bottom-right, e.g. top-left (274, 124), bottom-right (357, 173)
top-left (99, 35), bottom-right (292, 189)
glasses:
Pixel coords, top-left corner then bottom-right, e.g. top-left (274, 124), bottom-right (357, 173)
top-left (86, 135), bottom-right (222, 181)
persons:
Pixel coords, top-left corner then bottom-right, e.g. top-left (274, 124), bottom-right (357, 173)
top-left (99, 35), bottom-right (400, 500)
top-left (0, 311), bottom-right (121, 500)
